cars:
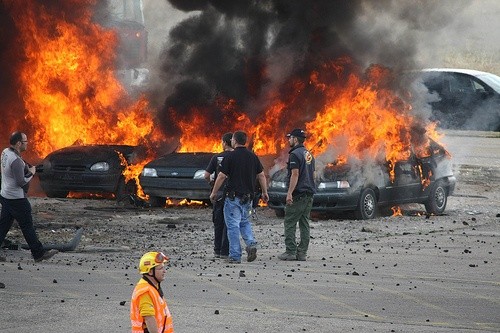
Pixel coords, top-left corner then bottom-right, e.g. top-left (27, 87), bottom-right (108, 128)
top-left (397, 68), bottom-right (500, 132)
top-left (35, 139), bottom-right (220, 209)
top-left (266, 126), bottom-right (458, 221)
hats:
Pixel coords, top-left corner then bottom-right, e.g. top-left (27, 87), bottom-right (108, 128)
top-left (285, 128), bottom-right (306, 140)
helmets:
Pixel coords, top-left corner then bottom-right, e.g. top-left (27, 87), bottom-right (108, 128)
top-left (139, 251), bottom-right (169, 274)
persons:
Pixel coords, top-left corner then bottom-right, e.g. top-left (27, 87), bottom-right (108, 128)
top-left (278, 128), bottom-right (316, 260)
top-left (205, 133), bottom-right (234, 258)
top-left (130, 252), bottom-right (174, 333)
top-left (0, 132), bottom-right (58, 261)
top-left (210, 131), bottom-right (269, 264)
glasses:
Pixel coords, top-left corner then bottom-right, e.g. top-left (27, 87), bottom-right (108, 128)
top-left (22, 139), bottom-right (29, 144)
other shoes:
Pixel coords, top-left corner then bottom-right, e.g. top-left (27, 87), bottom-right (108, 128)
top-left (222, 258), bottom-right (240, 264)
top-left (0, 252), bottom-right (7, 261)
top-left (297, 251), bottom-right (307, 261)
top-left (34, 250), bottom-right (60, 263)
top-left (278, 250), bottom-right (296, 261)
top-left (213, 254), bottom-right (229, 258)
top-left (245, 244), bottom-right (257, 261)
